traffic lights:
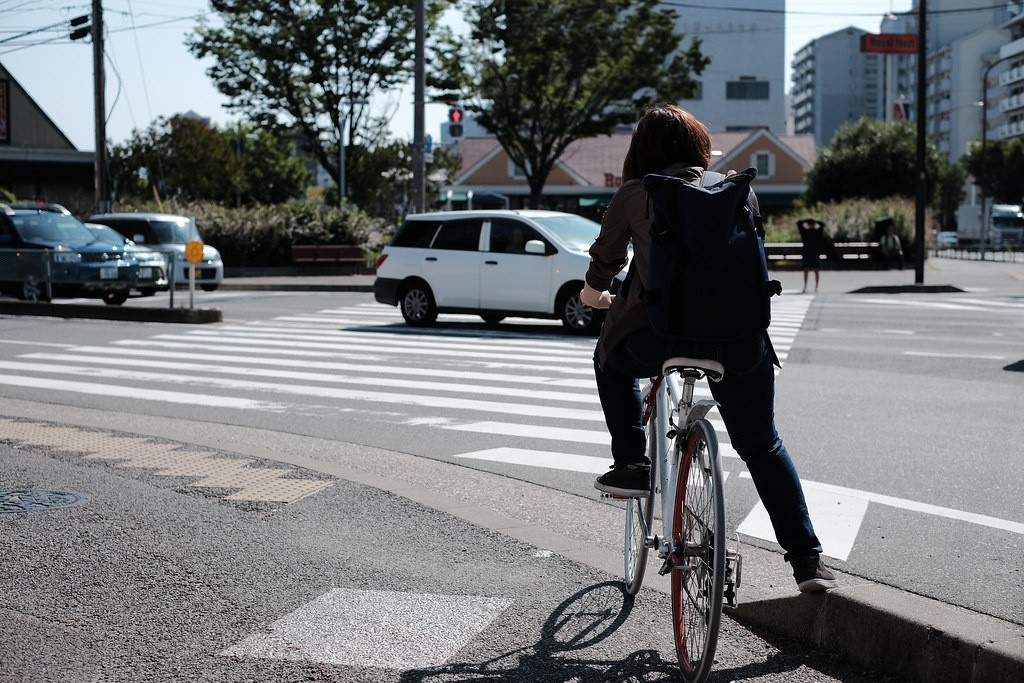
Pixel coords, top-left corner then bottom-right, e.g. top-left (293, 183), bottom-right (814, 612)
top-left (448, 107), bottom-right (466, 142)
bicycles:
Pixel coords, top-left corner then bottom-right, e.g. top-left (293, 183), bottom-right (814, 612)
top-left (583, 293), bottom-right (743, 683)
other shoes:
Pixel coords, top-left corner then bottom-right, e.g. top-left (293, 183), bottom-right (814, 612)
top-left (784, 548), bottom-right (839, 593)
top-left (594, 462), bottom-right (652, 499)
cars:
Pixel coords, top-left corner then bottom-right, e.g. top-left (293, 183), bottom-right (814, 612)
top-left (935, 231), bottom-right (960, 251)
top-left (81, 222), bottom-right (171, 295)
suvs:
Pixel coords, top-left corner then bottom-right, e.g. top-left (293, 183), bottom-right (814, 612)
top-left (375, 208), bottom-right (634, 337)
top-left (0, 201), bottom-right (140, 316)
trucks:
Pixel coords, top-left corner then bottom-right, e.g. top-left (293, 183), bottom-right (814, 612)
top-left (957, 204), bottom-right (1024, 252)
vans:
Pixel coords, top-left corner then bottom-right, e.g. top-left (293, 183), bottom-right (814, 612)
top-left (90, 212), bottom-right (224, 292)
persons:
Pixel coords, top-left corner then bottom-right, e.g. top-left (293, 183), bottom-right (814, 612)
top-left (579, 106), bottom-right (838, 594)
top-left (882, 226), bottom-right (903, 270)
top-left (796, 218), bottom-right (826, 294)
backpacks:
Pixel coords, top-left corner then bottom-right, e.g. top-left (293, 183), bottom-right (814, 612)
top-left (640, 167), bottom-right (782, 343)
